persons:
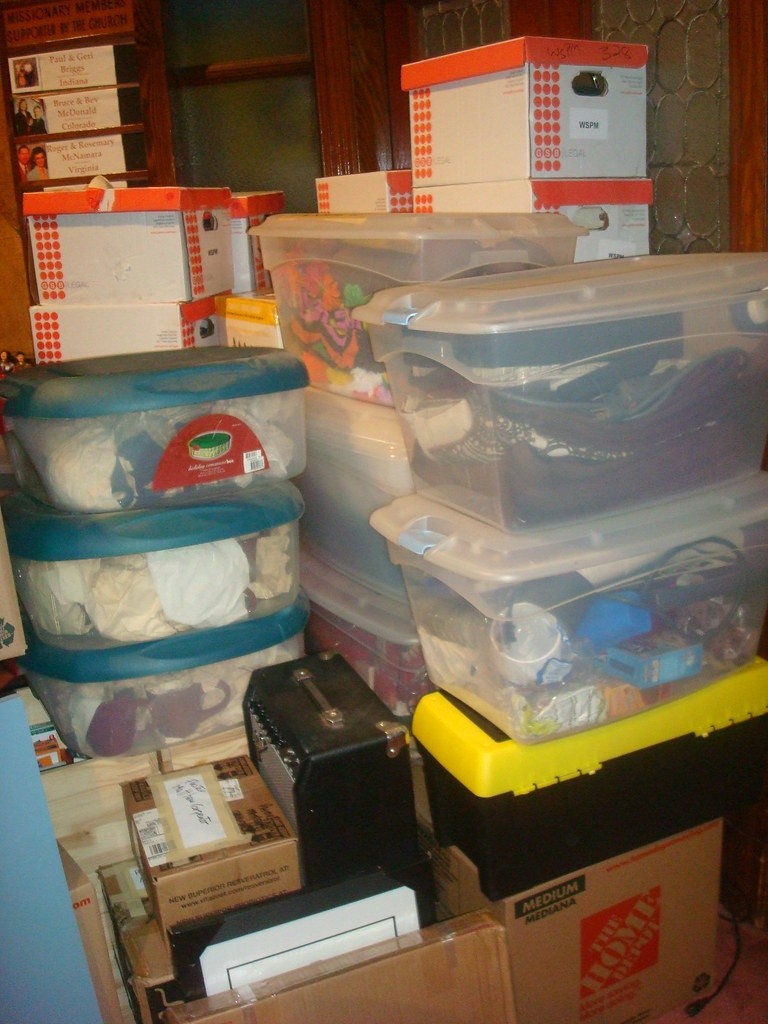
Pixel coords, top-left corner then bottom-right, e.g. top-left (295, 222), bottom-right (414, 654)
top-left (12, 62), bottom-right (49, 179)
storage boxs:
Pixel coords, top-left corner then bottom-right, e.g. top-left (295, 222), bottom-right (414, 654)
top-left (2, 38), bottom-right (766, 1024)
